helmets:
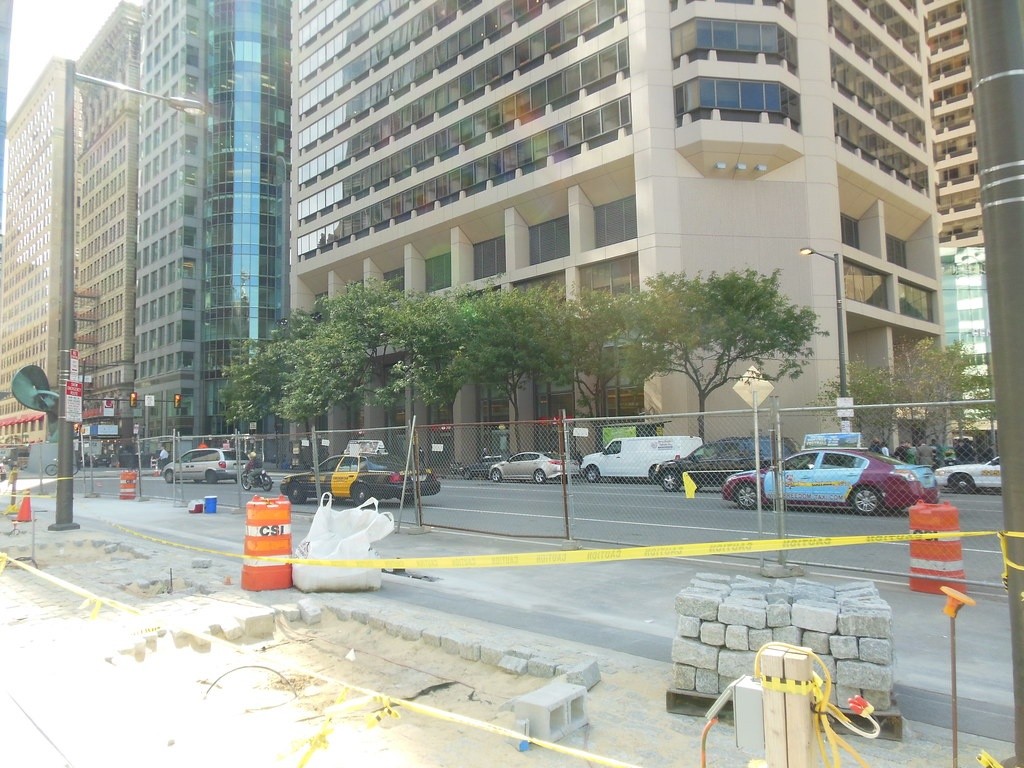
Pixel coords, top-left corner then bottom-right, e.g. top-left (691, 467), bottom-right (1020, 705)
top-left (250, 452), bottom-right (256, 457)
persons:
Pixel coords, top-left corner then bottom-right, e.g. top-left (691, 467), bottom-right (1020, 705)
top-left (245, 451), bottom-right (263, 487)
top-left (480, 447), bottom-right (490, 462)
top-left (865, 435), bottom-right (980, 474)
top-left (157, 446), bottom-right (170, 477)
top-left (417, 446), bottom-right (426, 466)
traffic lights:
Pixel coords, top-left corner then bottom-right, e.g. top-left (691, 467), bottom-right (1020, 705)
top-left (130, 392), bottom-right (137, 407)
top-left (174, 393), bottom-right (182, 408)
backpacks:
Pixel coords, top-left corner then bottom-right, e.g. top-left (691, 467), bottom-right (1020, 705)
top-left (254, 458), bottom-right (262, 468)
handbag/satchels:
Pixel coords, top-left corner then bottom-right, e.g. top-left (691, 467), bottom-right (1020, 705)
top-left (289, 493), bottom-right (395, 592)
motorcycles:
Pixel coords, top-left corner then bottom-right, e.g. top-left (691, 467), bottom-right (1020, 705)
top-left (240, 466), bottom-right (273, 492)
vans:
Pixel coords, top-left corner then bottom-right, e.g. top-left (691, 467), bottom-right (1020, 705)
top-left (160, 447), bottom-right (250, 483)
top-left (579, 435), bottom-right (704, 482)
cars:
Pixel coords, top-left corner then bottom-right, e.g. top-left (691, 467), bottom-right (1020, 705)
top-left (722, 431), bottom-right (939, 516)
top-left (487, 450), bottom-right (582, 484)
top-left (934, 454), bottom-right (1002, 495)
top-left (280, 439), bottom-right (442, 507)
top-left (458, 455), bottom-right (506, 479)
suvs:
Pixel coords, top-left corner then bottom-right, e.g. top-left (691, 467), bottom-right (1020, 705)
top-left (652, 436), bottom-right (800, 492)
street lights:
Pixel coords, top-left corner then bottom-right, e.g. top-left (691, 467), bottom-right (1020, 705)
top-left (961, 254), bottom-right (997, 458)
top-left (47, 58), bottom-right (208, 532)
top-left (799, 246), bottom-right (851, 433)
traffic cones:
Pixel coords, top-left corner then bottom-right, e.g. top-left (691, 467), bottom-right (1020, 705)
top-left (12, 489), bottom-right (33, 522)
